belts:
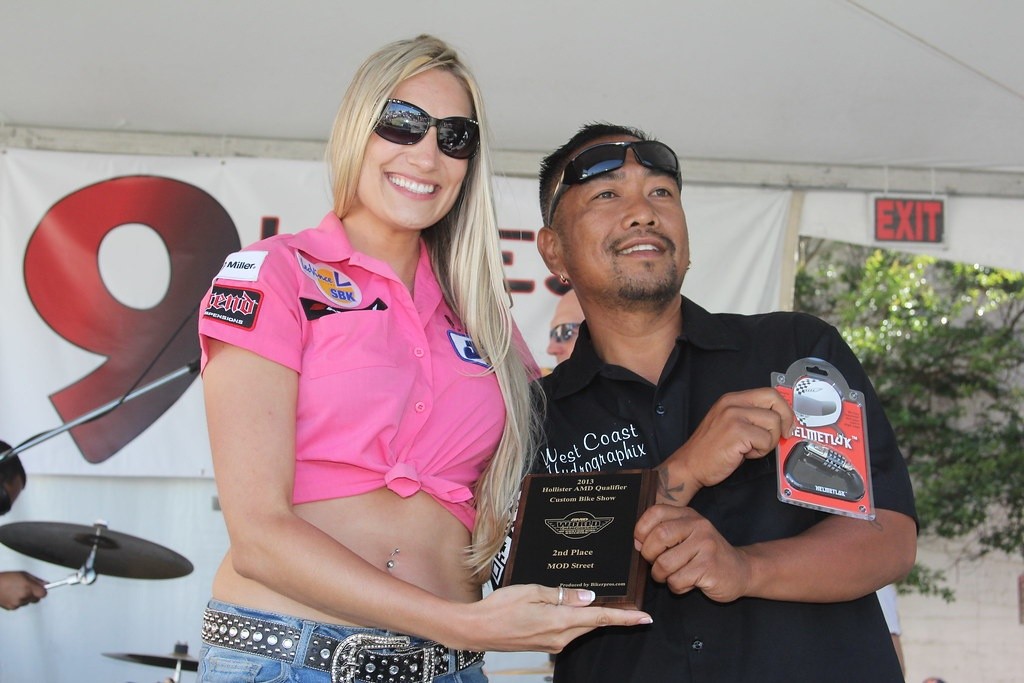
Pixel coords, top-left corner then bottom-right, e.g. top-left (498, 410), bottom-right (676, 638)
top-left (195, 608), bottom-right (489, 683)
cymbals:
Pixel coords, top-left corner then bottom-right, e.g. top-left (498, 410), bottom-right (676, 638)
top-left (0, 521), bottom-right (194, 580)
top-left (100, 652), bottom-right (199, 672)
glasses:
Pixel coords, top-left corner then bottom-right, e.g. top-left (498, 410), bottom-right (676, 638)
top-left (546, 135), bottom-right (684, 235)
top-left (547, 320), bottom-right (582, 343)
top-left (369, 93), bottom-right (480, 162)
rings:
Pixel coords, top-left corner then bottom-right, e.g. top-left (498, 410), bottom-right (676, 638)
top-left (558, 586), bottom-right (564, 604)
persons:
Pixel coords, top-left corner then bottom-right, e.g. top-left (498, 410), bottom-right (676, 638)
top-left (2, 446), bottom-right (51, 611)
top-left (199, 32), bottom-right (654, 683)
top-left (489, 122), bottom-right (919, 683)
